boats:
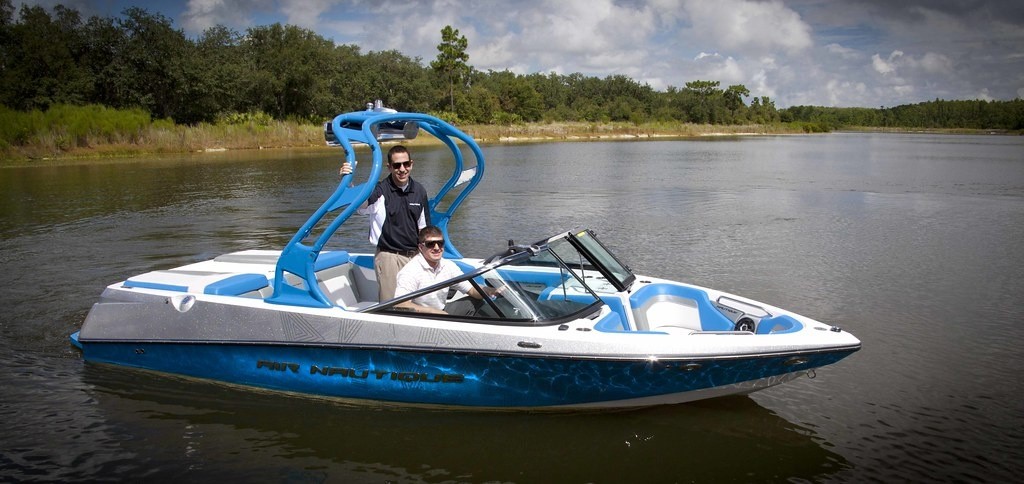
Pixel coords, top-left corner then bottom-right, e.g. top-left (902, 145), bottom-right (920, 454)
top-left (58, 101), bottom-right (862, 409)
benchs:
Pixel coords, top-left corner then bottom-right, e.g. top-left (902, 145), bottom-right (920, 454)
top-left (236, 263), bottom-right (382, 315)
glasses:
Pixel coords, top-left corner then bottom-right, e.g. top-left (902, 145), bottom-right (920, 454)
top-left (391, 161), bottom-right (412, 169)
top-left (422, 240), bottom-right (445, 248)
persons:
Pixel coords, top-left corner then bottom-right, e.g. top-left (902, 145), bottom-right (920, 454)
top-left (392, 227), bottom-right (508, 322)
top-left (339, 145), bottom-right (435, 313)
